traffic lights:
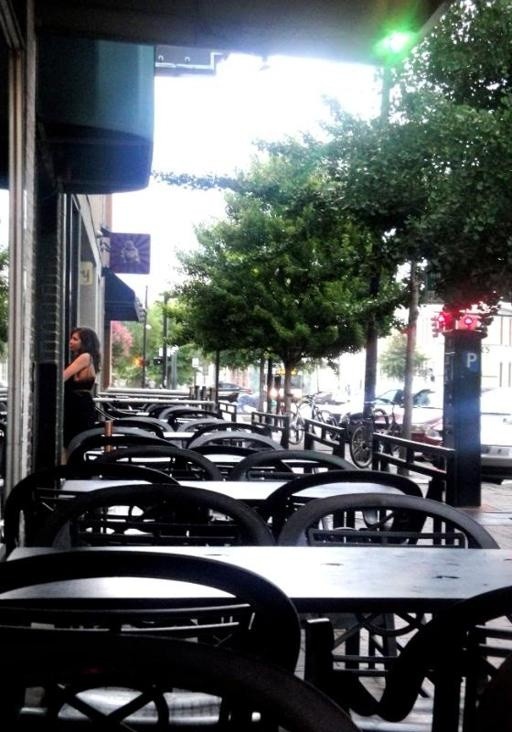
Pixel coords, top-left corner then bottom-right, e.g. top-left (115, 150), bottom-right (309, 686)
top-left (132, 357), bottom-right (164, 368)
top-left (431, 310), bottom-right (493, 339)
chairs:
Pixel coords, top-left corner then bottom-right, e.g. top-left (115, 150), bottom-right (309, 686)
top-left (0, 389), bottom-right (512, 732)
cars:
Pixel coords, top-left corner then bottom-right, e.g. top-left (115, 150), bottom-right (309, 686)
top-left (217, 382), bottom-right (251, 393)
top-left (326, 386), bottom-right (512, 482)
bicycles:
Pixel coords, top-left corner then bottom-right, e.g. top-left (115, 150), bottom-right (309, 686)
top-left (283, 391), bottom-right (401, 469)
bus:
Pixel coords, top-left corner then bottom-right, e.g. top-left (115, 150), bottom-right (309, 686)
top-left (272, 368), bottom-right (303, 403)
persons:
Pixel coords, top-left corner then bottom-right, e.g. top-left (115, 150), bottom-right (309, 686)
top-left (61, 326), bottom-right (105, 463)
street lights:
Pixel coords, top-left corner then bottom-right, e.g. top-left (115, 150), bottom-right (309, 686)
top-left (162, 289), bottom-right (182, 388)
